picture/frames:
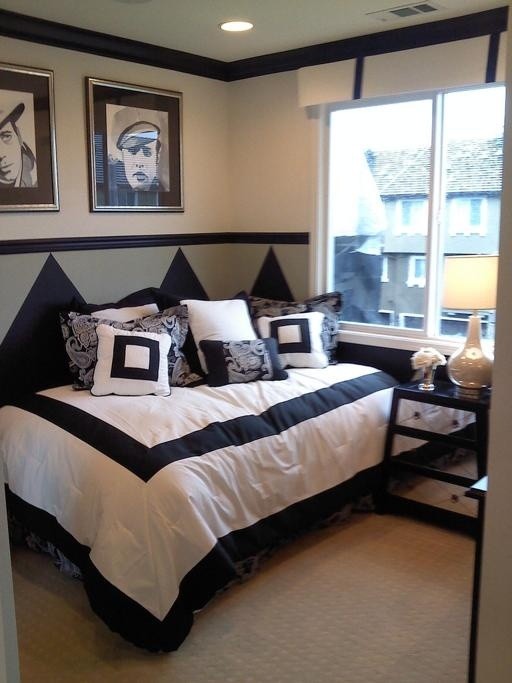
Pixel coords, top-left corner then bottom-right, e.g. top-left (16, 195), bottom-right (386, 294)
top-left (0, 61), bottom-right (60, 210)
top-left (84, 76), bottom-right (185, 212)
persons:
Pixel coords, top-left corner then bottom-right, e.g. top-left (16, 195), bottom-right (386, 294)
top-left (109, 108), bottom-right (168, 192)
top-left (1, 91), bottom-right (40, 187)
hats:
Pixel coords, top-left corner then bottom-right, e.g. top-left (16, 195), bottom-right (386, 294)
top-left (111, 107), bottom-right (164, 149)
top-left (0, 102), bottom-right (25, 130)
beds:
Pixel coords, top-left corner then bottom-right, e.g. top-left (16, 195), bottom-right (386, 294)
top-left (0, 359), bottom-right (477, 655)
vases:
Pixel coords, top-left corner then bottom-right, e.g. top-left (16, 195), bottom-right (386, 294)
top-left (418, 368), bottom-right (436, 392)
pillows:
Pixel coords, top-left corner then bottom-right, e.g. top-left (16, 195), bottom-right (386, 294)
top-left (257, 311), bottom-right (329, 371)
top-left (90, 324), bottom-right (173, 397)
top-left (198, 338), bottom-right (288, 386)
top-left (177, 298), bottom-right (262, 374)
top-left (242, 291), bottom-right (342, 366)
top-left (85, 297), bottom-right (159, 322)
top-left (58, 303), bottom-right (205, 390)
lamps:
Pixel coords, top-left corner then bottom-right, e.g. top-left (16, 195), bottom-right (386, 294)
top-left (443, 254), bottom-right (499, 390)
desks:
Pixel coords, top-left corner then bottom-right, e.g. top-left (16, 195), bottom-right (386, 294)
top-left (374, 386), bottom-right (490, 541)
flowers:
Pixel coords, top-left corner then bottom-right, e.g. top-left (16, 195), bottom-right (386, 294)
top-left (411, 346), bottom-right (447, 386)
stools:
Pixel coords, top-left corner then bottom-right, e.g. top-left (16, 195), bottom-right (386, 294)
top-left (463, 474), bottom-right (487, 683)
